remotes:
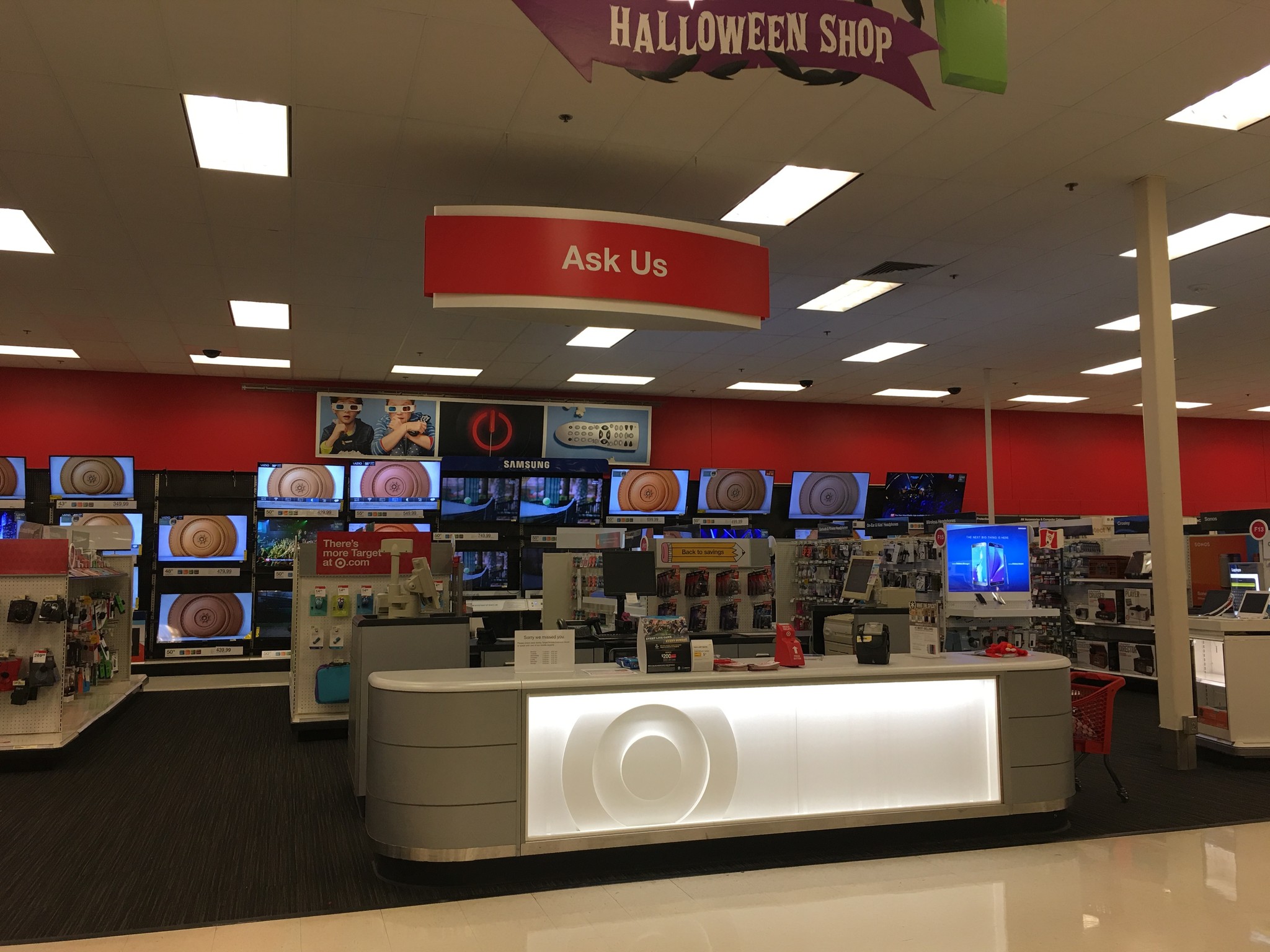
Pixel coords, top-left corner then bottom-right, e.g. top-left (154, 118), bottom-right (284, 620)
top-left (408, 414), bottom-right (431, 437)
top-left (554, 421), bottom-right (639, 451)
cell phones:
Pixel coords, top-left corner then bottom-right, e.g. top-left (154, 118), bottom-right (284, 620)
top-left (970, 542), bottom-right (1009, 587)
top-left (992, 592), bottom-right (1006, 605)
top-left (974, 594), bottom-right (987, 605)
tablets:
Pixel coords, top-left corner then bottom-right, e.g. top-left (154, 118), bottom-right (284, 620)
top-left (1236, 590), bottom-right (1270, 618)
top-left (1200, 590), bottom-right (1231, 617)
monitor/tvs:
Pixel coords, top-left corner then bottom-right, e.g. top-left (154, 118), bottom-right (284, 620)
top-left (346, 460), bottom-right (690, 609)
top-left (943, 522), bottom-right (1031, 602)
top-left (841, 555), bottom-right (882, 602)
top-left (254, 461), bottom-right (346, 639)
top-left (48, 455), bottom-right (143, 611)
top-left (697, 468), bottom-right (776, 538)
top-left (880, 472), bottom-right (968, 524)
top-left (0, 456), bottom-right (26, 540)
top-left (789, 471), bottom-right (870, 539)
top-left (155, 514), bottom-right (253, 646)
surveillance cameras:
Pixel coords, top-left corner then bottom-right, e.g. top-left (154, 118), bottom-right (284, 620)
top-left (799, 380), bottom-right (813, 388)
top-left (202, 350), bottom-right (221, 358)
top-left (948, 387), bottom-right (961, 395)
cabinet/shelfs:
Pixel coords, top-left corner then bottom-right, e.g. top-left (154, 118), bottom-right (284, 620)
top-left (1060, 513), bottom-right (1160, 692)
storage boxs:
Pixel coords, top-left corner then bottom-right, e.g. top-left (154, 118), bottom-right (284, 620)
top-left (1087, 587), bottom-right (1125, 625)
top-left (1076, 637), bottom-right (1120, 672)
top-left (1123, 587), bottom-right (1155, 627)
top-left (1117, 639), bottom-right (1158, 677)
top-left (1087, 552), bottom-right (1129, 579)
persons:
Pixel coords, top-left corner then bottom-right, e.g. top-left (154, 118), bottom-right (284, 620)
top-left (319, 396), bottom-right (374, 455)
top-left (371, 399), bottom-right (435, 456)
